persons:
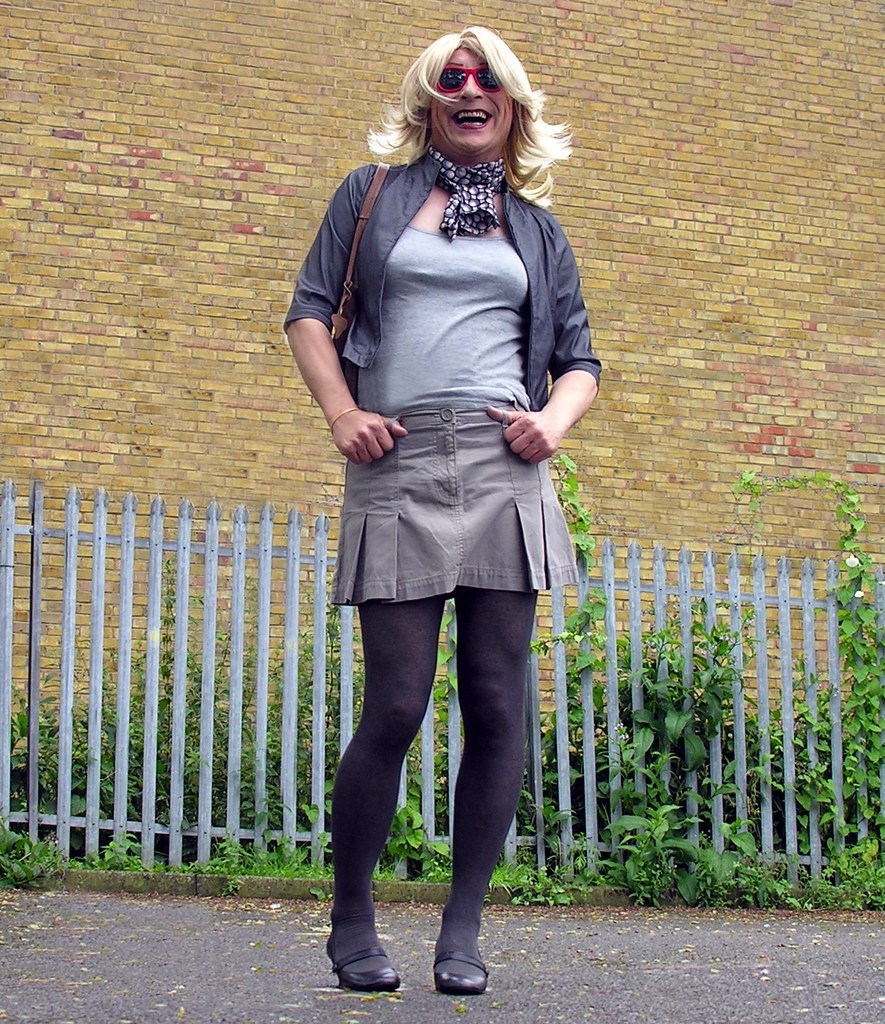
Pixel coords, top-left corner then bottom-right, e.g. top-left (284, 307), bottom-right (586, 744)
top-left (285, 25), bottom-right (605, 998)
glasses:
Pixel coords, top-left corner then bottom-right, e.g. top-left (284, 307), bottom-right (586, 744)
top-left (436, 66), bottom-right (503, 93)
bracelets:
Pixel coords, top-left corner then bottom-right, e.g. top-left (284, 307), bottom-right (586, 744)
top-left (328, 406), bottom-right (358, 432)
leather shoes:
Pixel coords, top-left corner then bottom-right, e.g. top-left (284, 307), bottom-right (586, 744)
top-left (433, 951), bottom-right (489, 994)
top-left (325, 935), bottom-right (400, 991)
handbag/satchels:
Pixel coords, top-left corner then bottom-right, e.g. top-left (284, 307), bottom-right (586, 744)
top-left (333, 163), bottom-right (392, 406)
